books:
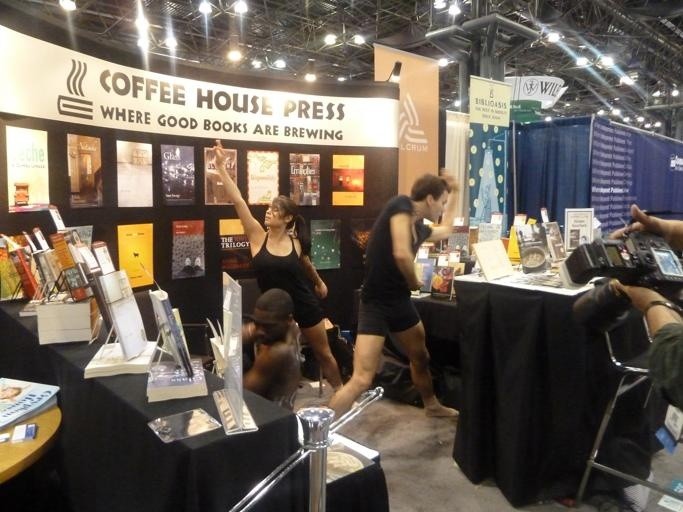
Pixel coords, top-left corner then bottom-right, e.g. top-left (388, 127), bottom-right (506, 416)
top-left (2, 225), bottom-right (207, 432)
top-left (411, 207), bottom-right (522, 302)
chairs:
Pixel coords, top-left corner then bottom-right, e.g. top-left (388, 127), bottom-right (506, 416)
top-left (133, 290), bottom-right (214, 370)
top-left (235, 278), bottom-right (325, 398)
top-left (573, 316), bottom-right (683, 508)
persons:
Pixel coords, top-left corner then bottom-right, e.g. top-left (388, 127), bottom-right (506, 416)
top-left (327, 167), bottom-right (461, 425)
top-left (212, 140), bottom-right (344, 394)
top-left (243, 288), bottom-right (301, 413)
top-left (611, 203), bottom-right (683, 413)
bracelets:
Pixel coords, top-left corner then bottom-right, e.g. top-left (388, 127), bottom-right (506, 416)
top-left (642, 301), bottom-right (683, 317)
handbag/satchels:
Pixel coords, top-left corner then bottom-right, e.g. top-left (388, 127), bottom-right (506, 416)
top-left (377, 361), bottom-right (424, 409)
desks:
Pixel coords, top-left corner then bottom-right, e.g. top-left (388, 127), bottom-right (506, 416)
top-left (451, 271), bottom-right (671, 508)
top-left (0, 403), bottom-right (62, 486)
top-left (353, 288), bottom-right (459, 409)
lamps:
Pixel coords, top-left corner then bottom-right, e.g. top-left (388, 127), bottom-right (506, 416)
top-left (386, 61), bottom-right (402, 83)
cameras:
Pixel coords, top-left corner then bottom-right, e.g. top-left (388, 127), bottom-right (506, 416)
top-left (559, 229), bottom-right (683, 339)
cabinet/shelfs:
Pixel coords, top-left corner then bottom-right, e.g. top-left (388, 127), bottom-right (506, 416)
top-left (0, 303), bottom-right (389, 512)
